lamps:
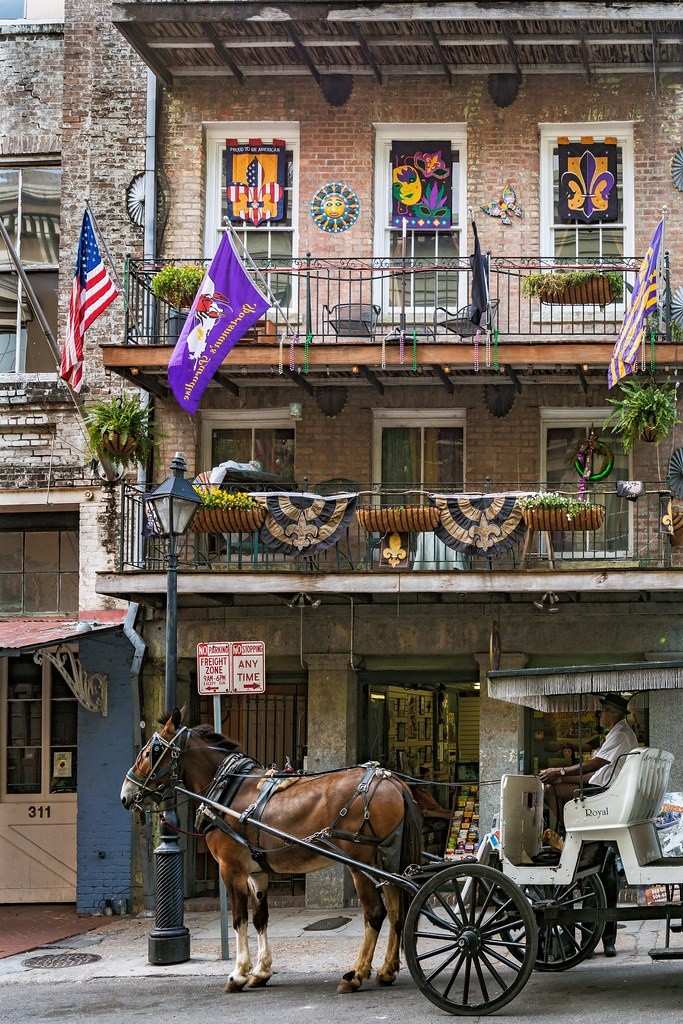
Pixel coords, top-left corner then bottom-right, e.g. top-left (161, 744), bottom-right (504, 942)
top-left (285, 593), bottom-right (321, 610)
top-left (288, 397), bottom-right (303, 421)
top-left (534, 591), bottom-right (560, 613)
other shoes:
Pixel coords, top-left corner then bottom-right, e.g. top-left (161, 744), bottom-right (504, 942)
top-left (604, 945), bottom-right (617, 957)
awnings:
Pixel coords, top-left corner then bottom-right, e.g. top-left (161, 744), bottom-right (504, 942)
top-left (0, 618), bottom-right (125, 657)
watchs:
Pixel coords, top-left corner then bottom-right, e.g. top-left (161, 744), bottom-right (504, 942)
top-left (561, 766), bottom-right (566, 776)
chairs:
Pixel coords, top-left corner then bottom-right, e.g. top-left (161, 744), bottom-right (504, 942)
top-left (302, 478), bottom-right (360, 571)
top-left (321, 301), bottom-right (381, 341)
top-left (434, 297), bottom-right (500, 341)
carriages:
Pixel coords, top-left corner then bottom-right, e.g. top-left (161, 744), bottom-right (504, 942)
top-left (118, 658), bottom-right (683, 1019)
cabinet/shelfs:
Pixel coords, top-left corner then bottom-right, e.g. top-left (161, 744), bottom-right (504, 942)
top-left (445, 783), bottom-right (481, 859)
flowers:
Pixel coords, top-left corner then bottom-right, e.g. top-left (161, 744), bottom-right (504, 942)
top-left (152, 262), bottom-right (204, 308)
top-left (519, 490), bottom-right (598, 521)
top-left (194, 484), bottom-right (257, 512)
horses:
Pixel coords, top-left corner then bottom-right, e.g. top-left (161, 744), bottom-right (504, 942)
top-left (120, 705), bottom-right (428, 994)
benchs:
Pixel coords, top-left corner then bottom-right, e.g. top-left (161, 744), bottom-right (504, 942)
top-left (564, 746), bottom-right (675, 831)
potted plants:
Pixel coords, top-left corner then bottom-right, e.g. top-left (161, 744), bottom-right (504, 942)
top-left (75, 388), bottom-right (170, 472)
top-left (602, 373), bottom-right (681, 457)
top-left (523, 270), bottom-right (623, 304)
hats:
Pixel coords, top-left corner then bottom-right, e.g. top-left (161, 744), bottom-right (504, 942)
top-left (599, 694), bottom-right (631, 715)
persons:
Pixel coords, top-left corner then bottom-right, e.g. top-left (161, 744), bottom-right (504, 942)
top-left (577, 842), bottom-right (620, 959)
top-left (539, 693), bottom-right (639, 824)
top-left (561, 743), bottom-right (580, 766)
top-left (412, 767), bottom-right (451, 830)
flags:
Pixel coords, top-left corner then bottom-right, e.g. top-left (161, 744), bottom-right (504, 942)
top-left (59, 203), bottom-right (119, 400)
top-left (167, 227), bottom-right (272, 415)
top-left (471, 228), bottom-right (490, 326)
top-left (607, 219), bottom-right (663, 390)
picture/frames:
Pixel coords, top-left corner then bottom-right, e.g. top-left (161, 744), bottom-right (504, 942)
top-left (397, 695), bottom-right (450, 771)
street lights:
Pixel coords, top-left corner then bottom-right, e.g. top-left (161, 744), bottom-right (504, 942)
top-left (143, 450), bottom-right (203, 967)
